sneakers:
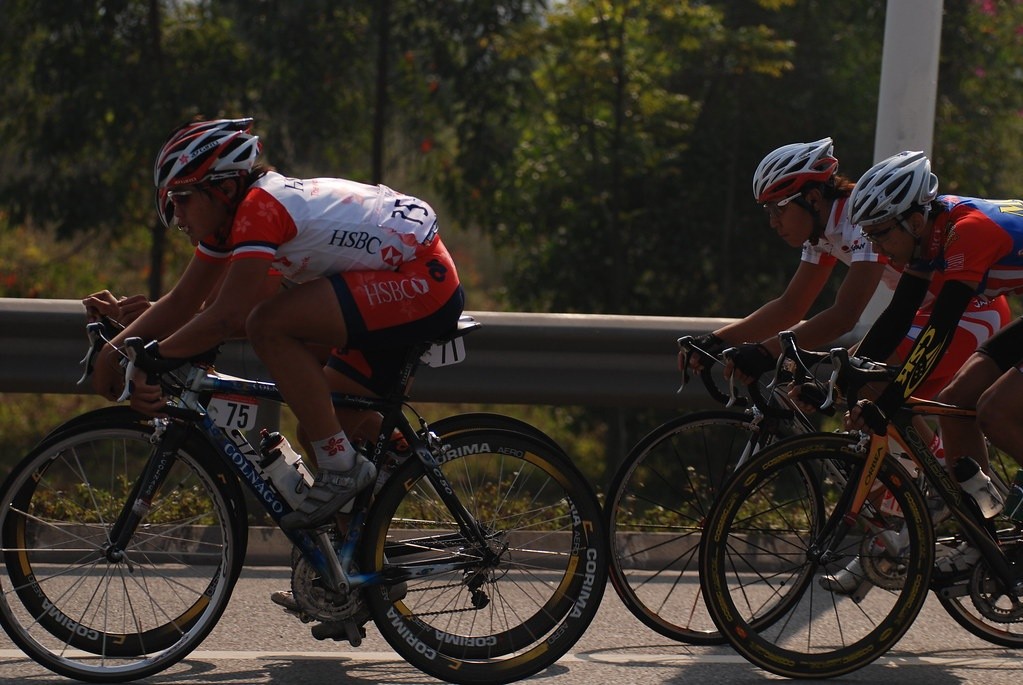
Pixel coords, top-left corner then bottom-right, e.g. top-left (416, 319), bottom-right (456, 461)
top-left (281, 450), bottom-right (377, 527)
top-left (311, 581), bottom-right (408, 640)
top-left (931, 541), bottom-right (993, 573)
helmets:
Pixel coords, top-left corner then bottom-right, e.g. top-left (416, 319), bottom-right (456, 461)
top-left (753, 136), bottom-right (837, 206)
top-left (845, 149), bottom-right (938, 226)
top-left (154, 116), bottom-right (262, 198)
top-left (152, 186), bottom-right (176, 229)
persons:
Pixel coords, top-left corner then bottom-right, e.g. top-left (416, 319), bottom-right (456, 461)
top-left (675, 134), bottom-right (1023, 603)
top-left (81, 116), bottom-right (466, 640)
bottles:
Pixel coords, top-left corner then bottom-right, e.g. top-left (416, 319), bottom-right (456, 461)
top-left (260, 428), bottom-right (316, 487)
top-left (337, 437), bottom-right (368, 514)
top-left (952, 455), bottom-right (1003, 519)
top-left (259, 447), bottom-right (311, 511)
top-left (1001, 466), bottom-right (1023, 524)
top-left (880, 452), bottom-right (919, 518)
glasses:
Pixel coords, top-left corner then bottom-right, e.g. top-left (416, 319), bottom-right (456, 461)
top-left (862, 215), bottom-right (914, 243)
top-left (166, 185), bottom-right (212, 204)
top-left (761, 192), bottom-right (805, 216)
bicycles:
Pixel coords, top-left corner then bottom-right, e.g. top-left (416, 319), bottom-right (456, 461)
top-left (604, 329), bottom-right (1023, 679)
top-left (1, 309), bottom-right (612, 685)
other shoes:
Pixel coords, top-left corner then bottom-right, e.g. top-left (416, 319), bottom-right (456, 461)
top-left (818, 541), bottom-right (890, 602)
top-left (271, 590), bottom-right (300, 613)
